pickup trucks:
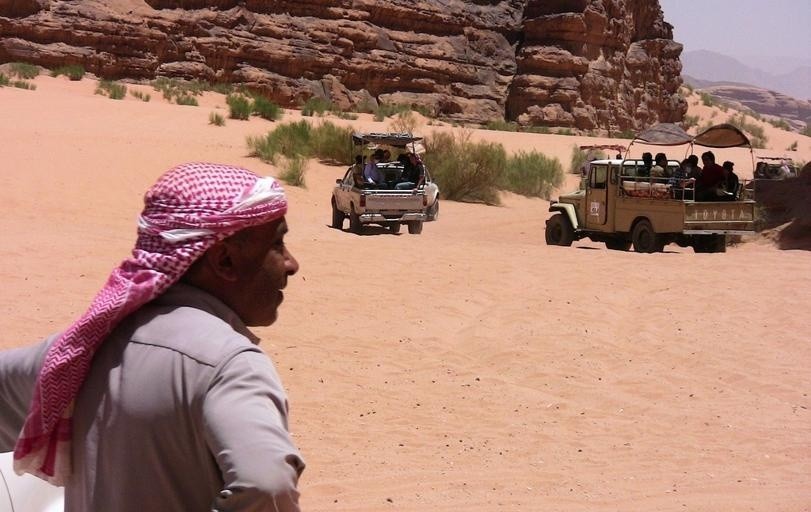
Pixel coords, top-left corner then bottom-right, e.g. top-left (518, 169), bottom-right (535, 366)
top-left (544, 156), bottom-right (757, 254)
top-left (329, 158), bottom-right (427, 237)
top-left (739, 161), bottom-right (802, 216)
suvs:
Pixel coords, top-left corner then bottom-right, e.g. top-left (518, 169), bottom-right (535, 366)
top-left (389, 160), bottom-right (440, 220)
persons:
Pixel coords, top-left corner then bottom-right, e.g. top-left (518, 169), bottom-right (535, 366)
top-left (1, 162), bottom-right (305, 512)
top-left (344, 141), bottom-right (431, 194)
top-left (629, 145), bottom-right (740, 202)
top-left (754, 160), bottom-right (792, 180)
